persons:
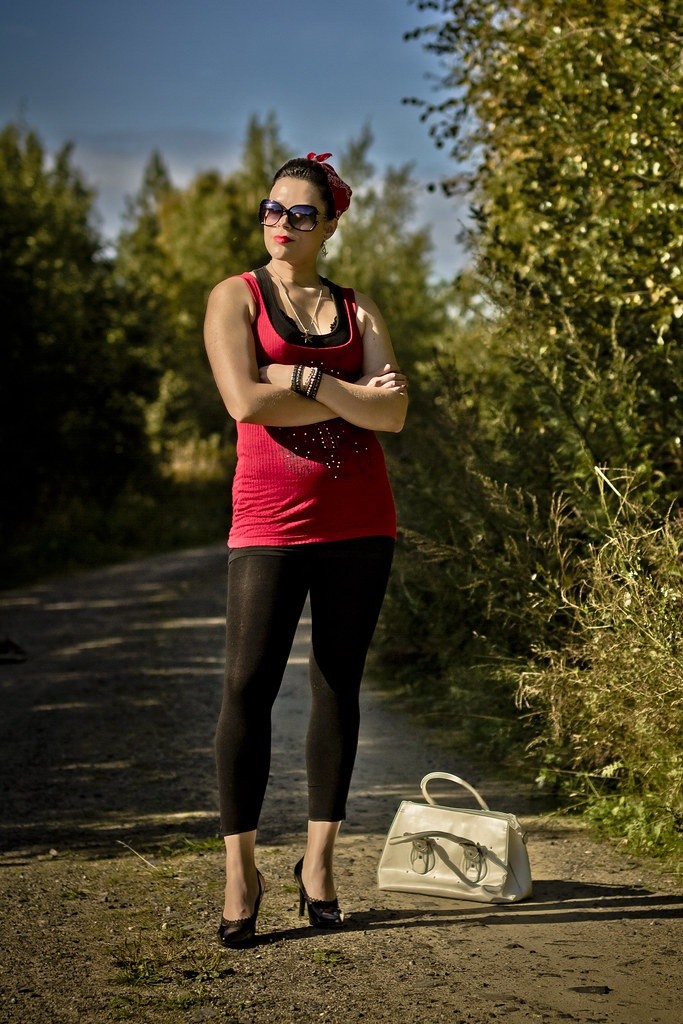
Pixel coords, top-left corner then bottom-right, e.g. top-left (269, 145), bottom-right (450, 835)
top-left (202, 150), bottom-right (410, 945)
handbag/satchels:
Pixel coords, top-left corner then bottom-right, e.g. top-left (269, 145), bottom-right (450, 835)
top-left (382, 772), bottom-right (533, 905)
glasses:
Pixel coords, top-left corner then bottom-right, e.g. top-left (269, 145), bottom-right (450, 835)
top-left (255, 198), bottom-right (330, 232)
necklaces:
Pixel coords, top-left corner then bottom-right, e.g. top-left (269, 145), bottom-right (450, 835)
top-left (268, 261), bottom-right (324, 343)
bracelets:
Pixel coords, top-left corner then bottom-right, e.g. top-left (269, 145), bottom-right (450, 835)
top-left (291, 365), bottom-right (325, 400)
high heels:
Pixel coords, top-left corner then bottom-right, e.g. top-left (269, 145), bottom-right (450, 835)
top-left (211, 865), bottom-right (266, 950)
top-left (288, 852), bottom-right (344, 925)
